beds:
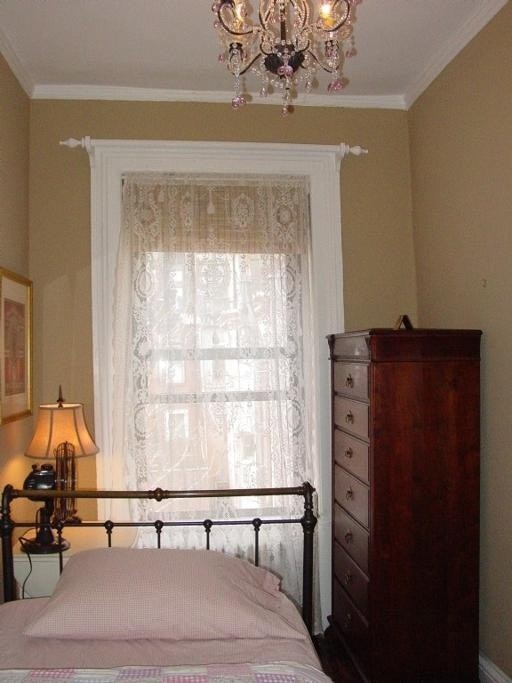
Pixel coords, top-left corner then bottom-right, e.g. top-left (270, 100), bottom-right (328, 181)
top-left (0, 481), bottom-right (325, 683)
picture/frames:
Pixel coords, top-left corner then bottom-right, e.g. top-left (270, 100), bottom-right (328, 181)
top-left (2, 266), bottom-right (37, 428)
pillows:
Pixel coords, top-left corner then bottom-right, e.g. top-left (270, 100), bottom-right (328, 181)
top-left (23, 546), bottom-right (307, 644)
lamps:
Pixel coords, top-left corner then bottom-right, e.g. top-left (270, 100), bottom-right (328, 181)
top-left (210, 0), bottom-right (359, 119)
top-left (24, 383), bottom-right (100, 527)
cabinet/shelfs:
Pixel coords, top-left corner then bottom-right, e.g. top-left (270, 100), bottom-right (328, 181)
top-left (326, 325), bottom-right (482, 678)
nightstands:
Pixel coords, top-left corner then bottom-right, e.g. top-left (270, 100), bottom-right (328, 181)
top-left (15, 520), bottom-right (140, 595)
